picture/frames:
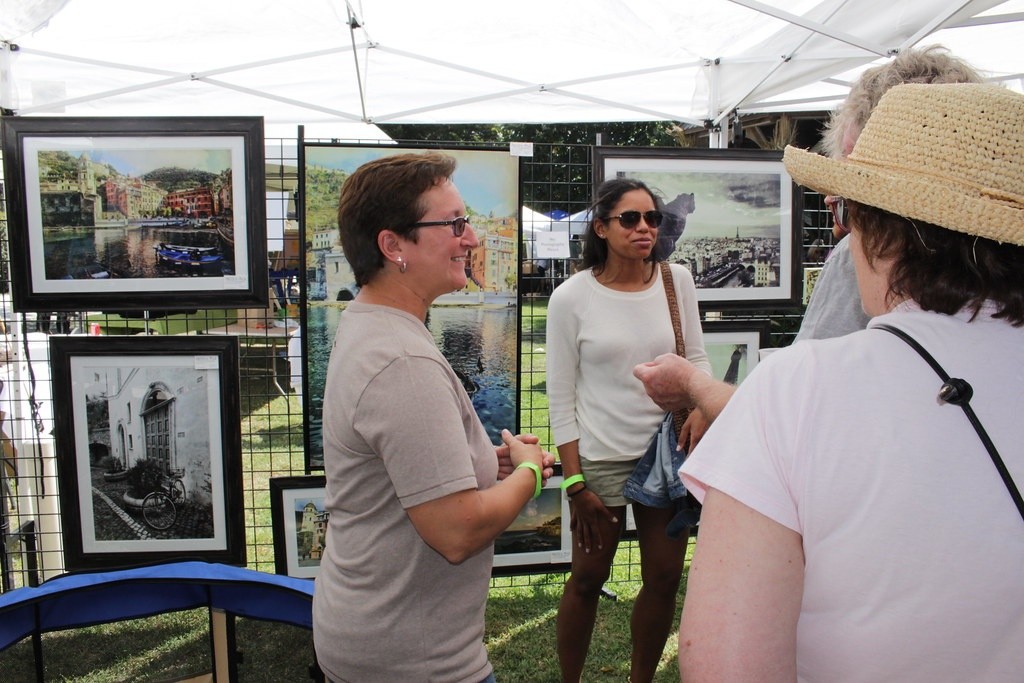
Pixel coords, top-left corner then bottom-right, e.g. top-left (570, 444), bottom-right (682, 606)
top-left (0, 115), bottom-right (269, 313)
top-left (297, 142), bottom-right (523, 477)
top-left (698, 319), bottom-right (771, 390)
top-left (49, 335), bottom-right (247, 573)
top-left (269, 465), bottom-right (572, 579)
top-left (594, 144), bottom-right (803, 310)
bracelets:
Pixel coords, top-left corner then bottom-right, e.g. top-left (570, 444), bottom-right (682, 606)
top-left (559, 474), bottom-right (586, 491)
top-left (517, 462), bottom-right (542, 500)
top-left (565, 486), bottom-right (584, 500)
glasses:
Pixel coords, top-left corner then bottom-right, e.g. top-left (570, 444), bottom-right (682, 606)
top-left (836, 196), bottom-right (861, 227)
top-left (607, 210), bottom-right (664, 229)
top-left (406, 214), bottom-right (470, 237)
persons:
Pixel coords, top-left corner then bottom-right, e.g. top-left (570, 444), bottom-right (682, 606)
top-left (630, 43), bottom-right (980, 424)
top-left (312, 152), bottom-right (556, 683)
top-left (676, 81), bottom-right (1024, 682)
top-left (546, 181), bottom-right (712, 682)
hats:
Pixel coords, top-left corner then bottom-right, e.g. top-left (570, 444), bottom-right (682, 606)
top-left (781, 82), bottom-right (1024, 246)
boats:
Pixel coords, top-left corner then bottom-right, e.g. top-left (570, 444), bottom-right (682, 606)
top-left (154, 243), bottom-right (221, 264)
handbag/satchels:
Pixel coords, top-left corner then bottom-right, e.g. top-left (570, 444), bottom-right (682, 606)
top-left (672, 406), bottom-right (691, 454)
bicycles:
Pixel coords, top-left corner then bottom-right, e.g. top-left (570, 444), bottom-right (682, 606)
top-left (142, 465), bottom-right (188, 530)
